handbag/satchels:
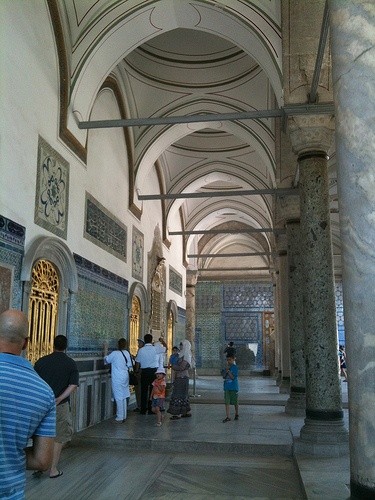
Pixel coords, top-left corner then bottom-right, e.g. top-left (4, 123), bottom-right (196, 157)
top-left (129, 371), bottom-right (138, 385)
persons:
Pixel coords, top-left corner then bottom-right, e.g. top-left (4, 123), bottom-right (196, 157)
top-left (221, 353), bottom-right (240, 423)
top-left (223, 342), bottom-right (236, 374)
top-left (33, 335), bottom-right (80, 479)
top-left (0, 309), bottom-right (56, 500)
top-left (338, 344), bottom-right (348, 382)
top-left (103, 334), bottom-right (193, 427)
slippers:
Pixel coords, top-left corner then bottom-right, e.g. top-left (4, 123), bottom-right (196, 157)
top-left (49, 468), bottom-right (63, 478)
top-left (32, 470), bottom-right (41, 477)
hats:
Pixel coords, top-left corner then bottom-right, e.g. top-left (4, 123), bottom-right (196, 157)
top-left (137, 338), bottom-right (145, 343)
top-left (228, 342), bottom-right (235, 345)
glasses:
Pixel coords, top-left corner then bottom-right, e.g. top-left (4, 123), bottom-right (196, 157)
top-left (173, 350), bottom-right (177, 351)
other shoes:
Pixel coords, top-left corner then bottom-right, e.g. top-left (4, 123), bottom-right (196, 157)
top-left (161, 415), bottom-right (165, 422)
top-left (155, 367), bottom-right (166, 374)
top-left (223, 417), bottom-right (231, 424)
top-left (170, 415), bottom-right (181, 419)
top-left (181, 412), bottom-right (192, 418)
top-left (155, 421), bottom-right (162, 427)
top-left (111, 419), bottom-right (126, 424)
top-left (234, 414), bottom-right (239, 421)
